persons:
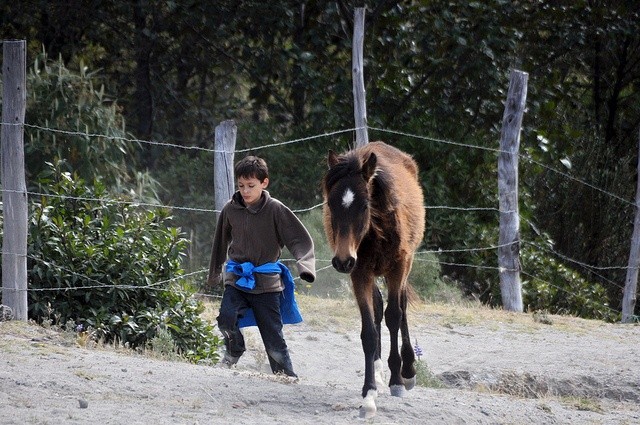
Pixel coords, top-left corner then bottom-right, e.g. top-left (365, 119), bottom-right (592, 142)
top-left (207, 155), bottom-right (317, 382)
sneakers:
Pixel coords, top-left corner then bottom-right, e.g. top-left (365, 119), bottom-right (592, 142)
top-left (220, 352), bottom-right (240, 371)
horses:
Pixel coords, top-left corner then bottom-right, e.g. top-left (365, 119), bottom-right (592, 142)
top-left (322, 141), bottom-right (426, 419)
